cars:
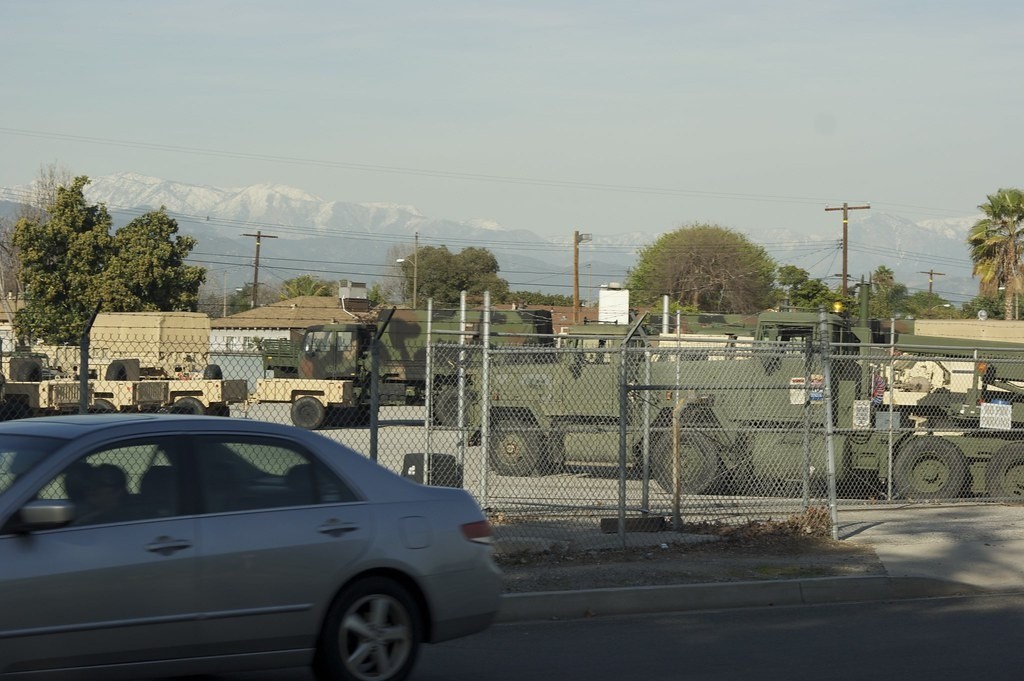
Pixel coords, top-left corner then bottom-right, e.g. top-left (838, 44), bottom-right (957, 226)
top-left (0, 408), bottom-right (503, 681)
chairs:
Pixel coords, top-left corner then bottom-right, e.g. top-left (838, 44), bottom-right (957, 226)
top-left (129, 467), bottom-right (184, 518)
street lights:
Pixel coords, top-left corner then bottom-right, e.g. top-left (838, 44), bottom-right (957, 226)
top-left (586, 284), bottom-right (607, 309)
top-left (395, 257), bottom-right (418, 309)
top-left (998, 286), bottom-right (1020, 320)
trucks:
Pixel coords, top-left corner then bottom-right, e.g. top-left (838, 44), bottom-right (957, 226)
top-left (0, 282), bottom-right (1024, 508)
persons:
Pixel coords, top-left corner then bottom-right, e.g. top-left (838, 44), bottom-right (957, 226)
top-left (68, 464), bottom-right (138, 528)
